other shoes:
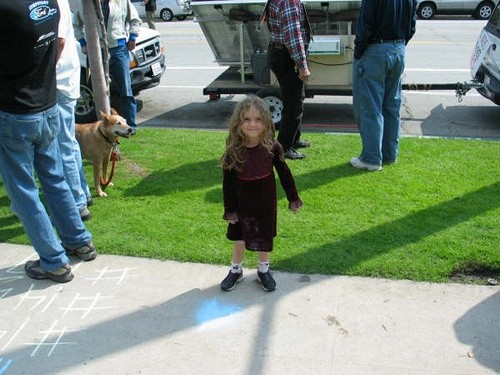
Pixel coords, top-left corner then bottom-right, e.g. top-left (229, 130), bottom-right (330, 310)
top-left (87, 196), bottom-right (93, 206)
top-left (79, 204), bottom-right (90, 220)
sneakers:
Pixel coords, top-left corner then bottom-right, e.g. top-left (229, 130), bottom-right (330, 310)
top-left (257, 269), bottom-right (276, 292)
top-left (282, 147), bottom-right (305, 159)
top-left (61, 241), bottom-right (97, 261)
top-left (297, 140), bottom-right (311, 149)
top-left (24, 259), bottom-right (74, 282)
top-left (351, 158), bottom-right (382, 170)
top-left (220, 267), bottom-right (244, 291)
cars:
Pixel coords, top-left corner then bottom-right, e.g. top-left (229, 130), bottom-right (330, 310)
top-left (414, 0), bottom-right (499, 21)
top-left (134, 0), bottom-right (195, 20)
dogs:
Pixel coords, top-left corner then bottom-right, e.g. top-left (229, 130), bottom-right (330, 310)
top-left (75, 108), bottom-right (133, 197)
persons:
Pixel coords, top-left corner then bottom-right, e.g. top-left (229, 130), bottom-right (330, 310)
top-left (0, 1), bottom-right (97, 283)
top-left (350, 0), bottom-right (416, 170)
top-left (265, 0), bottom-right (311, 160)
top-left (73, 0), bottom-right (142, 137)
top-left (56, 0), bottom-right (93, 220)
top-left (220, 94), bottom-right (303, 292)
top-left (143, 0), bottom-right (157, 30)
